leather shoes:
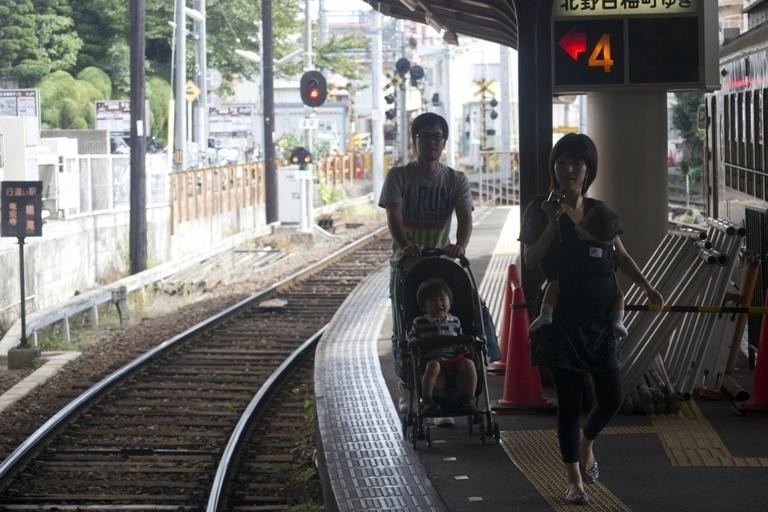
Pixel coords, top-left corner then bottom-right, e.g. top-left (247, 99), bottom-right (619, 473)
top-left (566, 482), bottom-right (589, 505)
top-left (581, 453), bottom-right (600, 484)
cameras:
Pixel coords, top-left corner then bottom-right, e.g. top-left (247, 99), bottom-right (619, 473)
top-left (547, 188), bottom-right (567, 208)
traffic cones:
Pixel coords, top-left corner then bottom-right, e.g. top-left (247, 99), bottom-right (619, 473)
top-left (667, 148), bottom-right (677, 168)
top-left (736, 297), bottom-right (767, 418)
top-left (489, 264), bottom-right (557, 417)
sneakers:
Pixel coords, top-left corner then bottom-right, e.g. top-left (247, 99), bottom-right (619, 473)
top-left (422, 394), bottom-right (441, 414)
top-left (427, 417), bottom-right (455, 426)
top-left (459, 394), bottom-right (479, 412)
top-left (398, 379), bottom-right (409, 413)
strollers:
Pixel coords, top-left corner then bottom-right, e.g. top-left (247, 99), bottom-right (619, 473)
top-left (389, 247), bottom-right (500, 449)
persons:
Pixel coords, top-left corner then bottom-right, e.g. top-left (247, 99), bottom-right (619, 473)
top-left (403, 275), bottom-right (478, 418)
top-left (513, 130), bottom-right (664, 504)
top-left (382, 108), bottom-right (395, 147)
top-left (528, 200), bottom-right (630, 339)
top-left (378, 112), bottom-right (476, 428)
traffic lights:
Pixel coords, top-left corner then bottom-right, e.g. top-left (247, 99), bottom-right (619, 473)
top-left (301, 71), bottom-right (327, 107)
